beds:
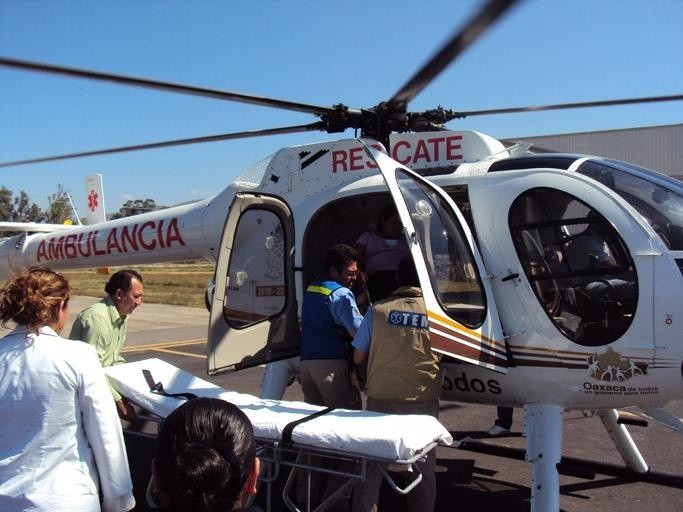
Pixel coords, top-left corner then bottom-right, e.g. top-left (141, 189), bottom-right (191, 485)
top-left (88, 352), bottom-right (452, 511)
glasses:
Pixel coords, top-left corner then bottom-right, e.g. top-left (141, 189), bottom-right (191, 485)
top-left (342, 271), bottom-right (360, 277)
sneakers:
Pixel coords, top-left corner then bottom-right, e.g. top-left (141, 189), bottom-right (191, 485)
top-left (487, 426), bottom-right (512, 435)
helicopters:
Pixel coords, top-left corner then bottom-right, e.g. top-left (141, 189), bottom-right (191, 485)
top-left (0, 0), bottom-right (683, 511)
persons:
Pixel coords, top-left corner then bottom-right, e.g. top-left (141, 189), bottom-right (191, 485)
top-left (0, 265), bottom-right (139, 512)
top-left (557, 214), bottom-right (616, 278)
top-left (351, 203), bottom-right (409, 294)
top-left (253, 353), bottom-right (325, 512)
top-left (67, 269), bottom-right (144, 416)
top-left (144, 397), bottom-right (262, 512)
top-left (487, 403), bottom-right (515, 437)
top-left (294, 243), bottom-right (366, 512)
top-left (351, 254), bottom-right (447, 512)
